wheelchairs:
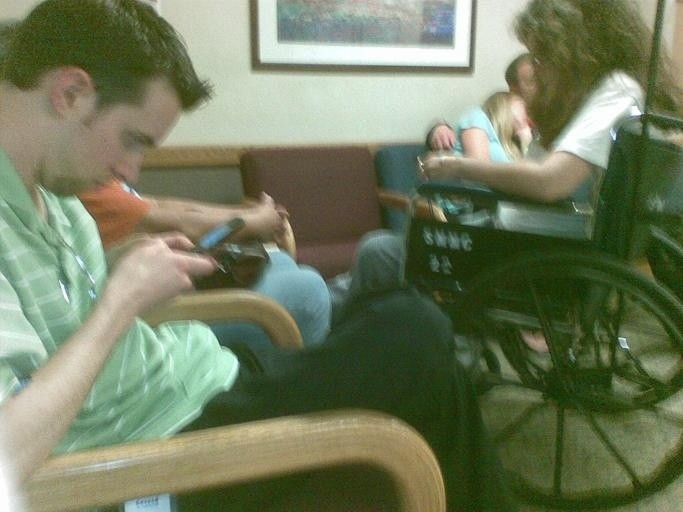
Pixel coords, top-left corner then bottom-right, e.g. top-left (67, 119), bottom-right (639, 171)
top-left (399, 113), bottom-right (683, 512)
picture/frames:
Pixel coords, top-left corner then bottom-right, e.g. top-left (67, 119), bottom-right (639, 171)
top-left (249, 0), bottom-right (476, 72)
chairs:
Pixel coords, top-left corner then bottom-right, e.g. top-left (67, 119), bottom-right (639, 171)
top-left (22, 284), bottom-right (446, 508)
top-left (241, 144), bottom-right (387, 282)
top-left (123, 152), bottom-right (246, 206)
top-left (373, 144), bottom-right (446, 239)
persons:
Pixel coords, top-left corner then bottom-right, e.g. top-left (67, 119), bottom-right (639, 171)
top-left (0, 0), bottom-right (546, 511)
top-left (328, 1), bottom-right (683, 327)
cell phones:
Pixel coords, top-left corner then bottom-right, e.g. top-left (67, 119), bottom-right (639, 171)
top-left (187, 218), bottom-right (246, 254)
